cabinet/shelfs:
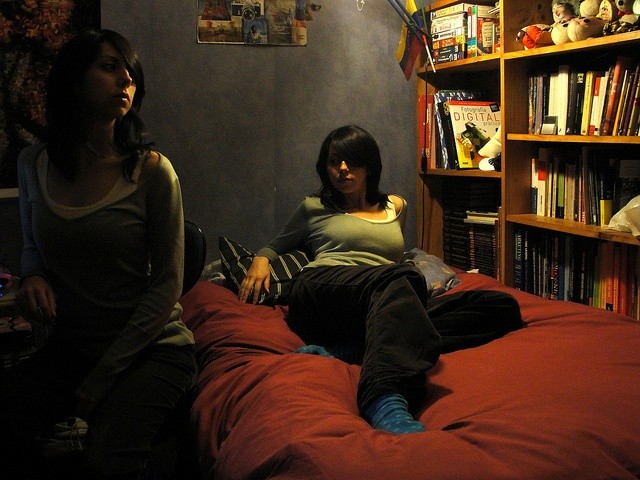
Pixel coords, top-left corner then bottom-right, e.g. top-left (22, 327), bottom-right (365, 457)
top-left (415, 0), bottom-right (503, 277)
top-left (499, 0), bottom-right (638, 318)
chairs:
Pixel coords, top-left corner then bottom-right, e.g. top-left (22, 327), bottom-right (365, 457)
top-left (184, 216), bottom-right (206, 296)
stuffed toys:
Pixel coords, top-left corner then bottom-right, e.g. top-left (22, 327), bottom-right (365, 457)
top-left (551, 0), bottom-right (615, 45)
top-left (516, 0), bottom-right (580, 49)
top-left (603, 0), bottom-right (640, 36)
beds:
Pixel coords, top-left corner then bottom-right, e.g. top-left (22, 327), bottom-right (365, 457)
top-left (185, 249), bottom-right (639, 479)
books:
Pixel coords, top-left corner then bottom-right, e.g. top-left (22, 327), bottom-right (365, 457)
top-left (433, 89), bottom-right (484, 171)
top-left (530, 145), bottom-right (640, 228)
top-left (512, 225), bottom-right (640, 318)
top-left (418, 95), bottom-right (434, 169)
top-left (472, 4), bottom-right (501, 58)
top-left (527, 62), bottom-right (640, 136)
top-left (467, 6), bottom-right (473, 60)
top-left (442, 204), bottom-right (501, 282)
top-left (428, 2), bottom-right (467, 64)
top-left (438, 100), bottom-right (501, 170)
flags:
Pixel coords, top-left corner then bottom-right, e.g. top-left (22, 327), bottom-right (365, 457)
top-left (396, 0), bottom-right (427, 82)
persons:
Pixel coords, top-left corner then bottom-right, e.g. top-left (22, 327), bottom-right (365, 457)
top-left (236, 124), bottom-right (520, 433)
top-left (247, 24), bottom-right (262, 40)
top-left (1, 28), bottom-right (196, 477)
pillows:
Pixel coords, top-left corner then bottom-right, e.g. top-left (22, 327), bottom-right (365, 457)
top-left (219, 235), bottom-right (324, 304)
